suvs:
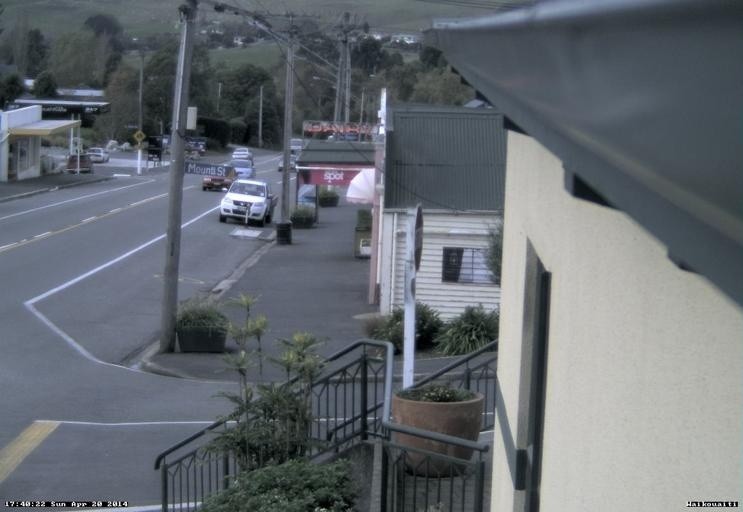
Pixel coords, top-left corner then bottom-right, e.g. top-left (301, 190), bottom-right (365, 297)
top-left (143, 135), bottom-right (204, 158)
top-left (289, 138), bottom-right (305, 153)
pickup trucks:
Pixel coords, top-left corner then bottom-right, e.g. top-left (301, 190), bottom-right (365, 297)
top-left (218, 178), bottom-right (277, 226)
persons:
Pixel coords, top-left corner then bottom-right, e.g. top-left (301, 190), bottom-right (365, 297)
top-left (256, 188), bottom-right (264, 196)
top-left (235, 186), bottom-right (248, 194)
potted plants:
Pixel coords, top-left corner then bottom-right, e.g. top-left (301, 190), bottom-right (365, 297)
top-left (289, 205), bottom-right (314, 229)
top-left (318, 185), bottom-right (339, 207)
top-left (175, 289), bottom-right (229, 352)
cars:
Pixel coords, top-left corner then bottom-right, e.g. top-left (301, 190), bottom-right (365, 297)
top-left (65, 154), bottom-right (93, 175)
top-left (231, 147), bottom-right (252, 160)
top-left (201, 165), bottom-right (241, 190)
top-left (277, 155), bottom-right (298, 171)
top-left (226, 158), bottom-right (256, 182)
top-left (86, 147), bottom-right (110, 164)
top-left (184, 137), bottom-right (205, 144)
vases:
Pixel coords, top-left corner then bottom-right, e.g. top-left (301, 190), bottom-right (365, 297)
top-left (391, 390), bottom-right (484, 478)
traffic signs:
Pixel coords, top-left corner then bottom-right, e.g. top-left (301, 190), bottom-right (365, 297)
top-left (182, 162), bottom-right (229, 178)
